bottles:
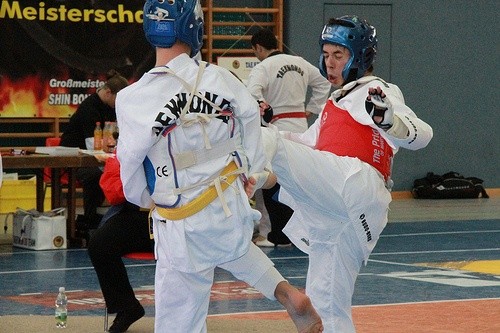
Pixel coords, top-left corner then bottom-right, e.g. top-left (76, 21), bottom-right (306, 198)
top-left (103, 121), bottom-right (119, 153)
top-left (94, 121), bottom-right (102, 150)
top-left (54, 287), bottom-right (68, 328)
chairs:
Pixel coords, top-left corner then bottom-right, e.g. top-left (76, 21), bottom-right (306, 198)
top-left (44, 137), bottom-right (82, 201)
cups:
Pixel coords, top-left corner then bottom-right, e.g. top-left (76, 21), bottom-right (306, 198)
top-left (85, 138), bottom-right (94, 150)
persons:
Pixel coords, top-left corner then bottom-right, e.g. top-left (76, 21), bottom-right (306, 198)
top-left (250, 9), bottom-right (434, 333)
top-left (58, 77), bottom-right (129, 244)
top-left (242, 28), bottom-right (332, 244)
top-left (88, 141), bottom-right (147, 333)
top-left (113, 0), bottom-right (329, 332)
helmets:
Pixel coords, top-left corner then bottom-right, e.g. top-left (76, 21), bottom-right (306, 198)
top-left (144, 0), bottom-right (205, 58)
top-left (318, 15), bottom-right (377, 83)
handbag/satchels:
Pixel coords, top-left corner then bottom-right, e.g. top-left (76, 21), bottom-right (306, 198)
top-left (3, 206), bottom-right (68, 250)
top-left (413, 172), bottom-right (489, 199)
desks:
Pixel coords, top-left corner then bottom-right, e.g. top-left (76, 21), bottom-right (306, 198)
top-left (2, 153), bottom-right (104, 248)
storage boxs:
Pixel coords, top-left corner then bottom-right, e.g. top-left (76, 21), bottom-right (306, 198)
top-left (12, 214), bottom-right (67, 250)
